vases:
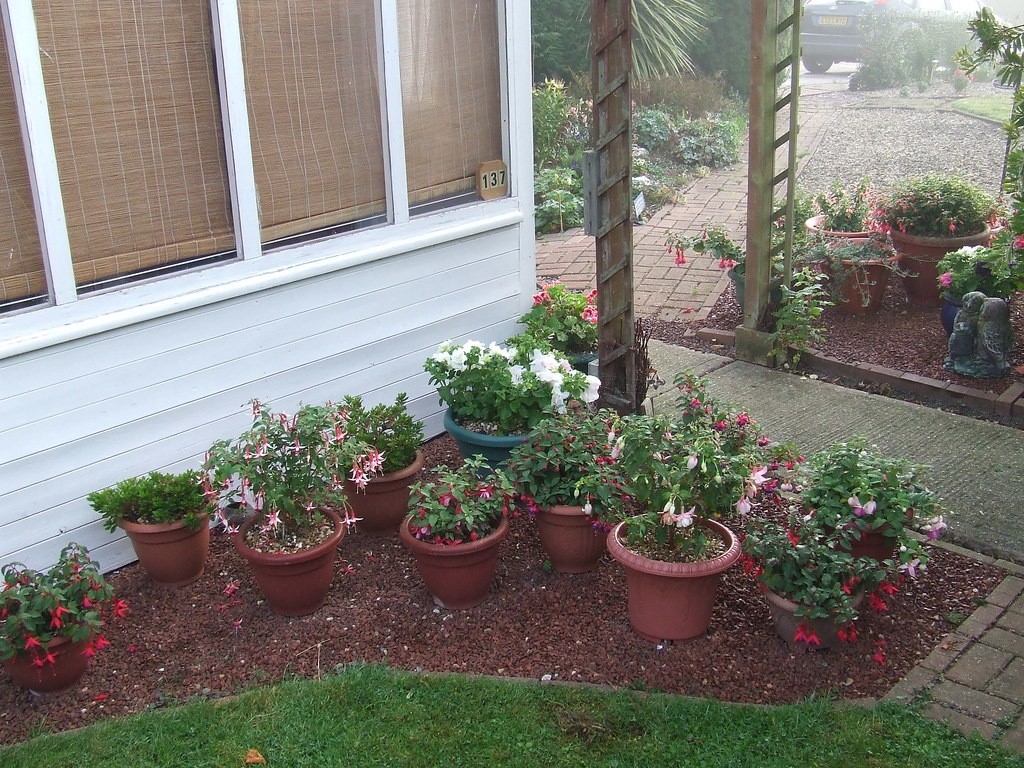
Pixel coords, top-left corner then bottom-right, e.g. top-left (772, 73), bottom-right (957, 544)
top-left (8, 621), bottom-right (92, 697)
top-left (823, 238), bottom-right (903, 318)
top-left (531, 504), bottom-right (611, 576)
top-left (444, 405), bottom-right (537, 483)
top-left (892, 220), bottom-right (992, 307)
top-left (727, 264), bottom-right (784, 312)
top-left (337, 448), bottom-right (425, 539)
top-left (762, 564), bottom-right (866, 651)
top-left (941, 283), bottom-right (1009, 339)
top-left (115, 509), bottom-right (210, 589)
top-left (606, 513), bottom-right (740, 641)
top-left (804, 215), bottom-right (879, 239)
top-left (828, 502), bottom-right (913, 569)
top-left (235, 506), bottom-right (344, 616)
top-left (401, 510), bottom-right (510, 610)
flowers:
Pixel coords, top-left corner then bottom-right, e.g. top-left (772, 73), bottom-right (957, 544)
top-left (663, 174), bottom-right (1023, 300)
top-left (1, 281), bottom-right (948, 667)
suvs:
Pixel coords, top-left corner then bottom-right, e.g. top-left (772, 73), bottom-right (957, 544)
top-left (799, 0), bottom-right (1015, 75)
